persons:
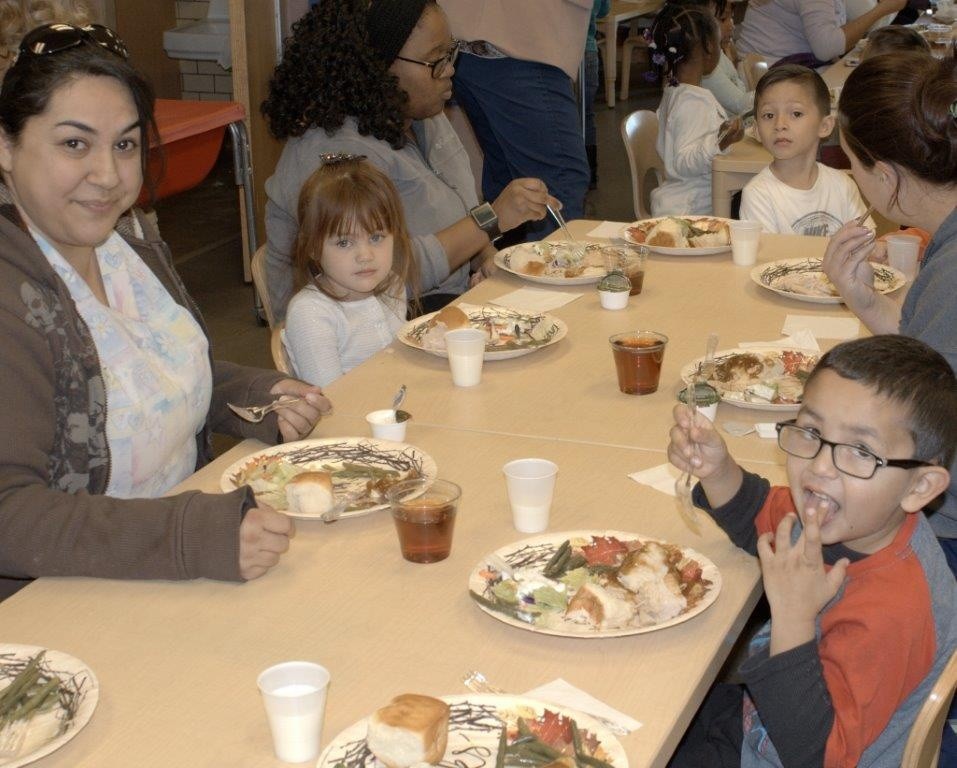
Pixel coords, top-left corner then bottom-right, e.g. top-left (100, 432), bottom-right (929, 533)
top-left (438, 1), bottom-right (596, 251)
top-left (1, 21), bottom-right (330, 601)
top-left (644, 3), bottom-right (745, 221)
top-left (585, 0), bottom-right (610, 189)
top-left (667, 0), bottom-right (758, 121)
top-left (284, 151), bottom-right (414, 391)
top-left (753, 25), bottom-right (931, 170)
top-left (740, 66), bottom-right (878, 239)
top-left (668, 334), bottom-right (957, 768)
top-left (735, 1), bottom-right (910, 78)
top-left (264, 0), bottom-right (562, 324)
top-left (753, 38), bottom-right (957, 619)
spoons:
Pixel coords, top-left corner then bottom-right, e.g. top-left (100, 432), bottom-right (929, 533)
top-left (548, 205), bottom-right (587, 260)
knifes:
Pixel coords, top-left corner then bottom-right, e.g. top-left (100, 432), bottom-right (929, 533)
top-left (696, 333), bottom-right (720, 383)
top-left (318, 488), bottom-right (370, 526)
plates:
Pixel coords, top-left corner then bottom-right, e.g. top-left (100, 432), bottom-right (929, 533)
top-left (469, 527), bottom-right (722, 639)
top-left (494, 240), bottom-right (641, 284)
top-left (317, 693), bottom-right (630, 768)
top-left (0, 643), bottom-right (99, 768)
top-left (395, 306), bottom-right (568, 362)
top-left (750, 257), bottom-right (907, 303)
top-left (622, 215), bottom-right (739, 256)
top-left (679, 345), bottom-right (825, 411)
top-left (220, 436), bottom-right (438, 522)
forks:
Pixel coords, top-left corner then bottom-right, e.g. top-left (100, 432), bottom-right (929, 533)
top-left (672, 382), bottom-right (703, 526)
top-left (227, 395), bottom-right (314, 423)
top-left (459, 667), bottom-right (628, 739)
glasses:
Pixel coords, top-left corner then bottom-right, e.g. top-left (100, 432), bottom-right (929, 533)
top-left (774, 418), bottom-right (932, 479)
top-left (399, 42), bottom-right (462, 78)
top-left (17, 23), bottom-right (130, 66)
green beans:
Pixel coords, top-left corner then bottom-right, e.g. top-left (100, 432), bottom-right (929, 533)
top-left (490, 712), bottom-right (617, 768)
top-left (1, 647), bottom-right (62, 731)
top-left (544, 539), bottom-right (585, 578)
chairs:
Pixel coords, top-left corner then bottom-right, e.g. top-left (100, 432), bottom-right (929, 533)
top-left (622, 108), bottom-right (670, 222)
top-left (269, 312), bottom-right (299, 384)
top-left (249, 246), bottom-right (279, 322)
top-left (896, 635), bottom-right (957, 768)
top-left (620, 34), bottom-right (673, 104)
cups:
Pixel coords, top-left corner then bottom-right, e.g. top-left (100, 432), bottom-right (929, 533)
top-left (503, 458), bottom-right (558, 535)
top-left (445, 328), bottom-right (489, 386)
top-left (384, 477), bottom-right (463, 564)
top-left (609, 331), bottom-right (668, 397)
top-left (258, 659), bottom-right (333, 763)
top-left (885, 234), bottom-right (923, 278)
top-left (726, 221), bottom-right (765, 266)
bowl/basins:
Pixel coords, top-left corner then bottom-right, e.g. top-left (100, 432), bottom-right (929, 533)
top-left (365, 409), bottom-right (413, 443)
top-left (596, 281), bottom-right (632, 309)
top-left (677, 388), bottom-right (720, 422)
top-left (927, 24), bottom-right (955, 43)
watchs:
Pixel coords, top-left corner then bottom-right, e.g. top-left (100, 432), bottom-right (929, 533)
top-left (470, 201), bottom-right (503, 242)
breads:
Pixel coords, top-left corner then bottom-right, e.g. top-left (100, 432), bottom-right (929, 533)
top-left (365, 692), bottom-right (451, 767)
top-left (508, 246), bottom-right (547, 277)
top-left (284, 472), bottom-right (334, 514)
top-left (644, 221), bottom-right (690, 248)
top-left (430, 306), bottom-right (469, 331)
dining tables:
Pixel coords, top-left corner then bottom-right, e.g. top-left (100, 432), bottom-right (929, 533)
top-left (591, 1), bottom-right (671, 105)
top-left (708, 7), bottom-right (956, 219)
top-left (0, 217), bottom-right (923, 768)
